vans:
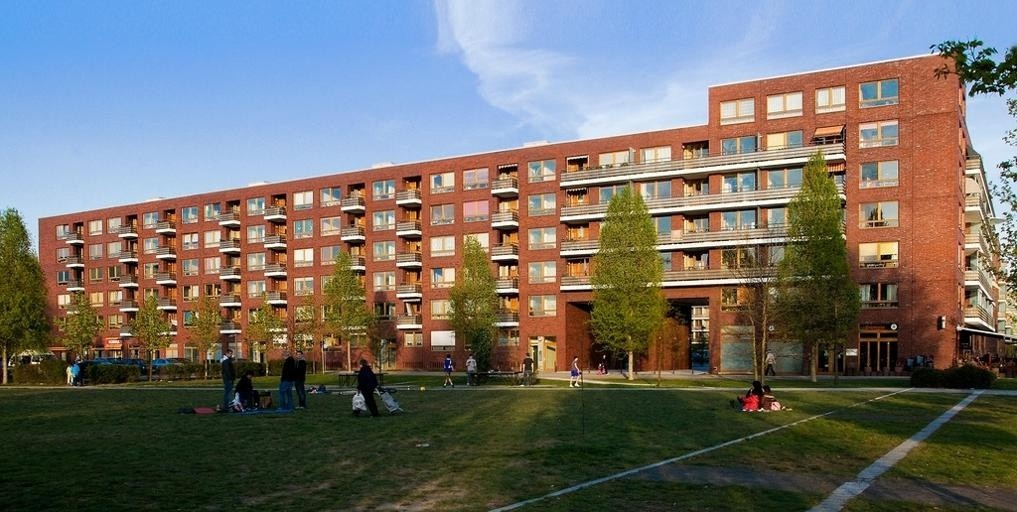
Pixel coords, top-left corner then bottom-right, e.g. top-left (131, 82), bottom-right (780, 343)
top-left (7, 350), bottom-right (56, 370)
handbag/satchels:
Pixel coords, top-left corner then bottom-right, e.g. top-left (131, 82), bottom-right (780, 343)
top-left (351, 394), bottom-right (366, 412)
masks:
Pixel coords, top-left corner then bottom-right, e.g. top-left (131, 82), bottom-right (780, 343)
top-left (360, 365), bottom-right (363, 368)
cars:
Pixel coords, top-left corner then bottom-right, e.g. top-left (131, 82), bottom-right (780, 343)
top-left (78, 355), bottom-right (192, 375)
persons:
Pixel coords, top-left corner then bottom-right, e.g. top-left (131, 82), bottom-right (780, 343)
top-left (353, 359), bottom-right (382, 418)
top-left (66, 360), bottom-right (83, 385)
top-left (235, 371), bottom-right (261, 411)
top-left (729, 379), bottom-right (776, 412)
top-left (602, 355), bottom-right (608, 374)
top-left (275, 351), bottom-right (295, 413)
top-left (465, 354), bottom-right (478, 386)
top-left (442, 352), bottom-right (456, 388)
top-left (520, 353), bottom-right (533, 386)
top-left (220, 348), bottom-right (233, 413)
top-left (294, 350), bottom-right (307, 410)
top-left (570, 356), bottom-right (581, 388)
top-left (765, 349), bottom-right (777, 376)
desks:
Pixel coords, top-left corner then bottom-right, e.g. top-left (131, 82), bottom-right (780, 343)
top-left (338, 372), bottom-right (389, 389)
top-left (466, 371), bottom-right (523, 386)
